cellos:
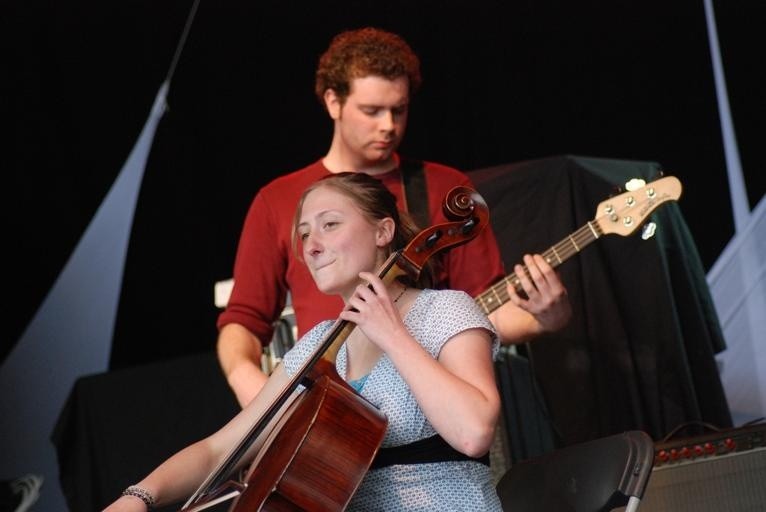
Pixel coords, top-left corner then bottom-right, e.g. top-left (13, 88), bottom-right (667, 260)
top-left (182, 187), bottom-right (489, 511)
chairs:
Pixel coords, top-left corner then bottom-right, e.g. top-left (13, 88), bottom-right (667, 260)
top-left (496, 430), bottom-right (656, 512)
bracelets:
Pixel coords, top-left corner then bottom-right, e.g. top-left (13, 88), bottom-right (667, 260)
top-left (122, 485), bottom-right (154, 511)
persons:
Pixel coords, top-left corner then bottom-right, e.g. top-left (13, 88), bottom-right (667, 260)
top-left (100, 172), bottom-right (503, 512)
top-left (216, 27), bottom-right (573, 409)
